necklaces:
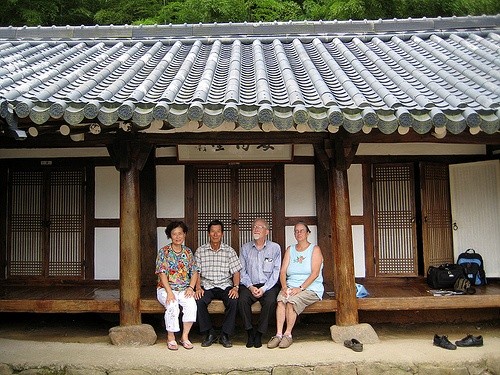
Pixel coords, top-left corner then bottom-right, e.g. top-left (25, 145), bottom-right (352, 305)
top-left (172, 248), bottom-right (182, 253)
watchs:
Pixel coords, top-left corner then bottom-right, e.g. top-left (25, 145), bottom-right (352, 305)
top-left (234, 285), bottom-right (239, 289)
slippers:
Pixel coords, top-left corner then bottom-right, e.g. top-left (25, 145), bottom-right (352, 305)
top-left (166, 338), bottom-right (178, 351)
top-left (179, 339), bottom-right (193, 349)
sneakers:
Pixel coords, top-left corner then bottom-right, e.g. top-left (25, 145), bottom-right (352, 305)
top-left (279, 334), bottom-right (292, 348)
top-left (455, 334), bottom-right (483, 346)
top-left (268, 334), bottom-right (282, 349)
top-left (433, 334), bottom-right (457, 350)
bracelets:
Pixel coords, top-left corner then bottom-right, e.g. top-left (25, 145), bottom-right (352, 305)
top-left (300, 285), bottom-right (304, 291)
top-left (189, 286), bottom-right (194, 291)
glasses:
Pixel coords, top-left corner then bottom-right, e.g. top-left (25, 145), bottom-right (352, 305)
top-left (253, 225), bottom-right (266, 230)
top-left (295, 231), bottom-right (306, 234)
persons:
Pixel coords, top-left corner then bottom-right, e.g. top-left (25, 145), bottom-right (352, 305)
top-left (155, 221), bottom-right (200, 350)
top-left (194, 220), bottom-right (243, 348)
top-left (238, 218), bottom-right (281, 348)
top-left (267, 221), bottom-right (324, 348)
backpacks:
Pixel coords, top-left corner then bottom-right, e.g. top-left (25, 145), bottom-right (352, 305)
top-left (459, 248), bottom-right (487, 287)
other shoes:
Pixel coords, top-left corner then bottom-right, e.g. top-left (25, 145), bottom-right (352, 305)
top-left (343, 339), bottom-right (363, 352)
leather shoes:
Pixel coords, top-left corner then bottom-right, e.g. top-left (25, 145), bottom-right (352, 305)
top-left (201, 335), bottom-right (218, 347)
top-left (219, 336), bottom-right (233, 348)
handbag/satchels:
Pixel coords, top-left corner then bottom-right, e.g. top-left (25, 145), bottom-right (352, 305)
top-left (427, 261), bottom-right (480, 289)
